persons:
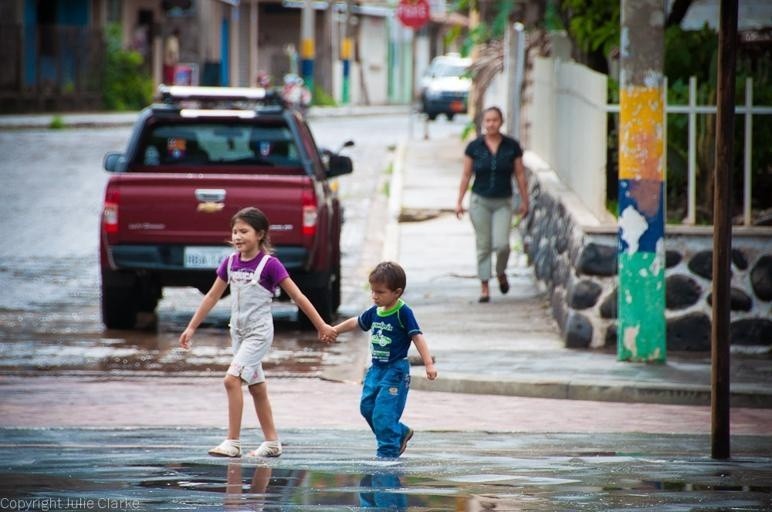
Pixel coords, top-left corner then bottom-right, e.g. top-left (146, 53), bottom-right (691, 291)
top-left (180, 207), bottom-right (338, 457)
top-left (225, 466), bottom-right (273, 510)
top-left (456, 106), bottom-right (529, 302)
top-left (322, 261), bottom-right (438, 458)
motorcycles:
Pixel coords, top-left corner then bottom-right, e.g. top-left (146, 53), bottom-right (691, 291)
top-left (317, 140), bottom-right (355, 196)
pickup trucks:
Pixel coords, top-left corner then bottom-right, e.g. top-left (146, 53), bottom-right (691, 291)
top-left (96, 82), bottom-right (358, 329)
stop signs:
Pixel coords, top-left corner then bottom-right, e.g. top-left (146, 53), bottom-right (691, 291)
top-left (397, 0), bottom-right (430, 28)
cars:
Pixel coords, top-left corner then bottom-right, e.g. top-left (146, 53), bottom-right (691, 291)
top-left (417, 52), bottom-right (478, 119)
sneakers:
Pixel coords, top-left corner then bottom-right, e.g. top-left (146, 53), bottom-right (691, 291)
top-left (399, 428), bottom-right (414, 456)
top-left (249, 440), bottom-right (283, 458)
top-left (208, 439), bottom-right (244, 458)
top-left (497, 273), bottom-right (508, 293)
top-left (480, 293), bottom-right (489, 303)
